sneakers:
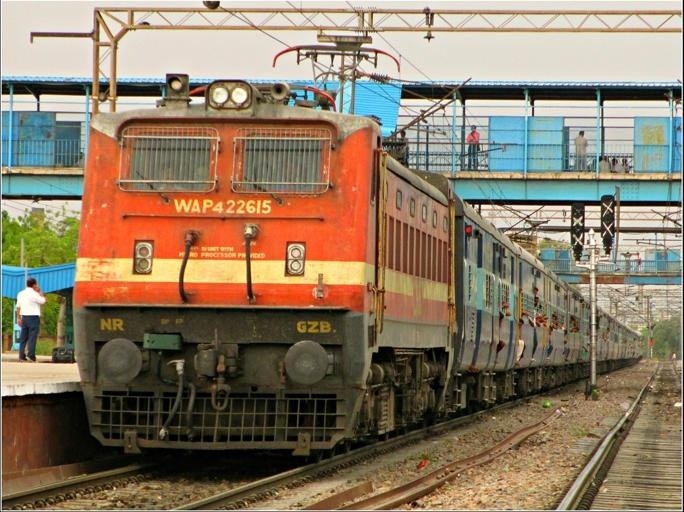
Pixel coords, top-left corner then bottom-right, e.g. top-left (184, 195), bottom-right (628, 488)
top-left (19, 356), bottom-right (28, 361)
top-left (27, 353), bottom-right (36, 360)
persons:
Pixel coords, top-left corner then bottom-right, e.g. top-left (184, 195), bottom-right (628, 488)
top-left (502, 285), bottom-right (579, 333)
top-left (589, 155), bottom-right (629, 173)
top-left (400, 130), bottom-right (409, 169)
top-left (574, 130), bottom-right (589, 171)
top-left (466, 124), bottom-right (480, 171)
top-left (15, 277), bottom-right (47, 362)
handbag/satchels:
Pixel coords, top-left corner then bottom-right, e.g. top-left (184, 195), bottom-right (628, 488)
top-left (53, 346), bottom-right (73, 363)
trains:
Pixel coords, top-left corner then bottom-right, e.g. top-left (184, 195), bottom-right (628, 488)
top-left (73, 73), bottom-right (644, 462)
